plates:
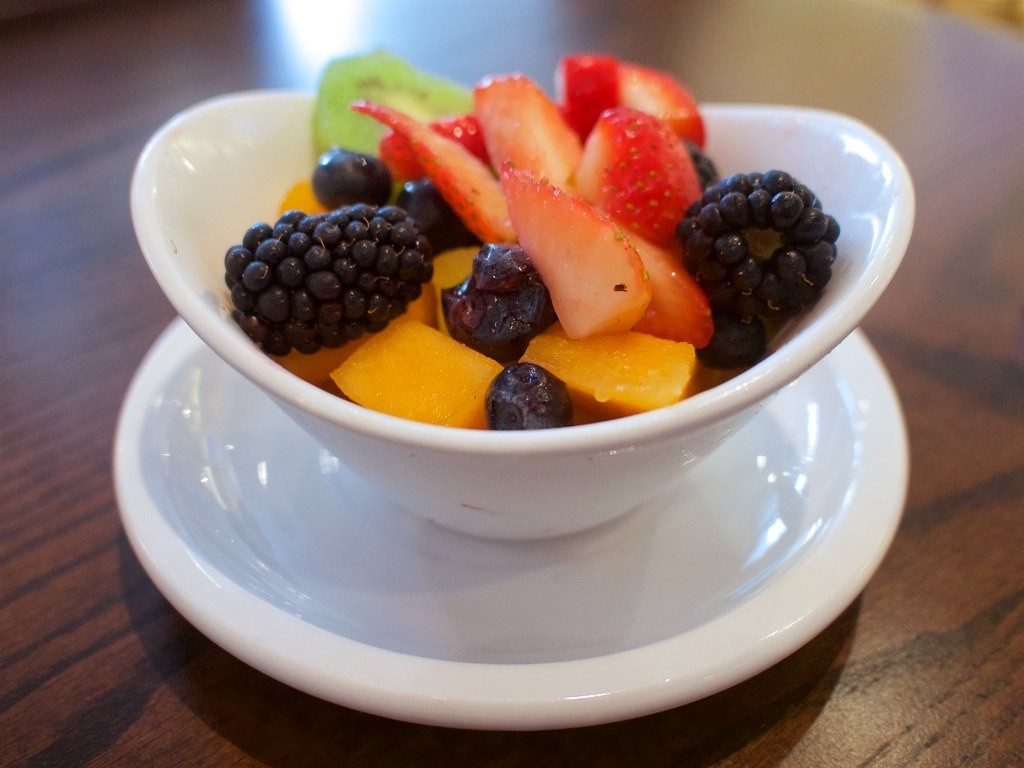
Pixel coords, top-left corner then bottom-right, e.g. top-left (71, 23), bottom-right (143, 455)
top-left (111, 317), bottom-right (910, 731)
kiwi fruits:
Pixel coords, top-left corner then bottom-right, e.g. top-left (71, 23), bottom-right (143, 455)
top-left (310, 51), bottom-right (478, 191)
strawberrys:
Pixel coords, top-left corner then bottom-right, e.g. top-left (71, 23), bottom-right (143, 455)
top-left (345, 53), bottom-right (712, 359)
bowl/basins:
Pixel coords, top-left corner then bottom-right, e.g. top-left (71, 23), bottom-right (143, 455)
top-left (128, 86), bottom-right (917, 540)
